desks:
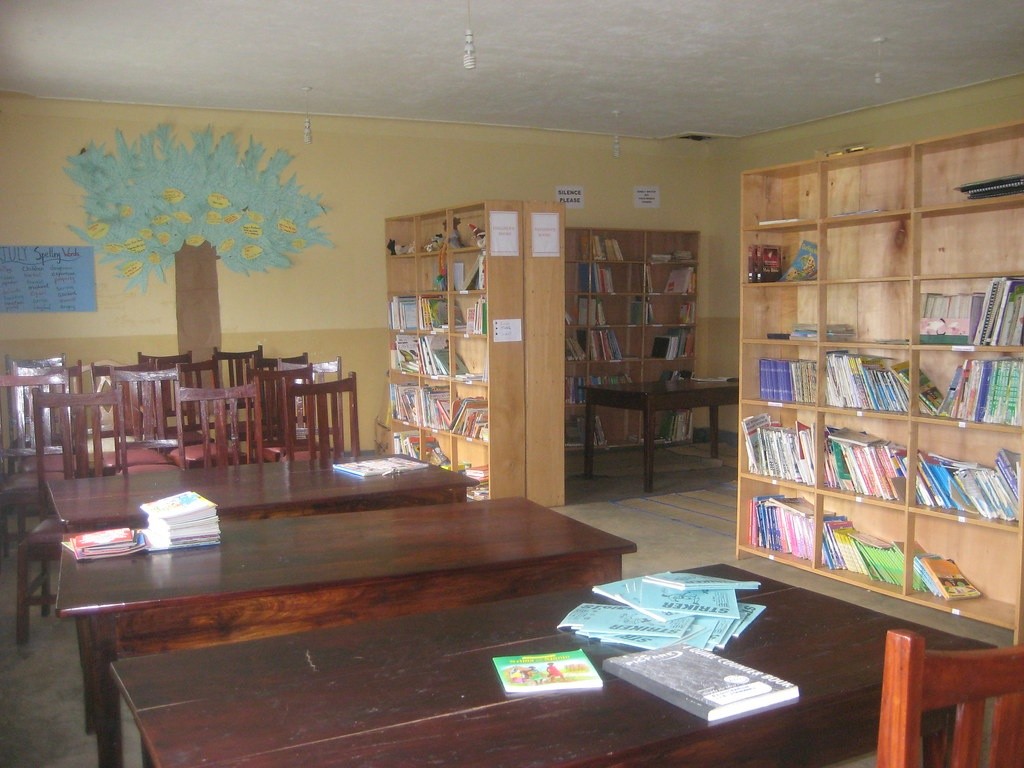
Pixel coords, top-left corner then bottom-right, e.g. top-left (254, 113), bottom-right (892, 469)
top-left (108, 564), bottom-right (1000, 768)
top-left (56, 495), bottom-right (638, 768)
top-left (579, 380), bottom-right (738, 493)
top-left (46, 457), bottom-right (480, 671)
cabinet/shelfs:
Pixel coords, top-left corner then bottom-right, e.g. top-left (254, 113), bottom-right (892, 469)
top-left (735, 120), bottom-right (1023, 648)
top-left (384, 201), bottom-right (699, 510)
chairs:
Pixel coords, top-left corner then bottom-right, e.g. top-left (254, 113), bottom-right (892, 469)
top-left (1, 344), bottom-right (361, 646)
top-left (877, 627), bottom-right (1024, 768)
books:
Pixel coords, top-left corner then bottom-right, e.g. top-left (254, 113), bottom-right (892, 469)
top-left (333, 249), bottom-right (489, 502)
top-left (491, 569), bottom-right (800, 722)
top-left (563, 236), bottom-right (695, 449)
top-left (758, 173), bottom-right (1024, 225)
top-left (748, 240), bottom-right (1024, 347)
top-left (60, 490), bottom-right (221, 561)
top-left (741, 349), bottom-right (1024, 601)
